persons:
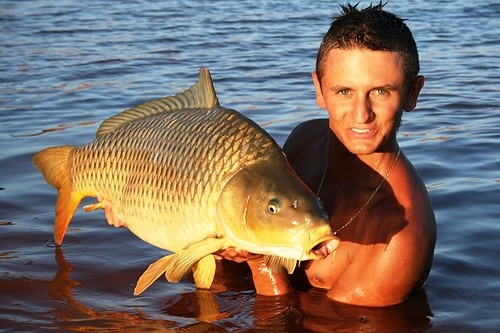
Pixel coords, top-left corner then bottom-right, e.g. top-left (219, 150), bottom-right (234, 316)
top-left (97, 0), bottom-right (438, 308)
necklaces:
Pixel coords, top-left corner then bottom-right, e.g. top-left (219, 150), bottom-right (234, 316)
top-left (315, 125), bottom-right (402, 237)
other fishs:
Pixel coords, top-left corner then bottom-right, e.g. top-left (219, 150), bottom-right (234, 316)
top-left (33, 67), bottom-right (341, 297)
